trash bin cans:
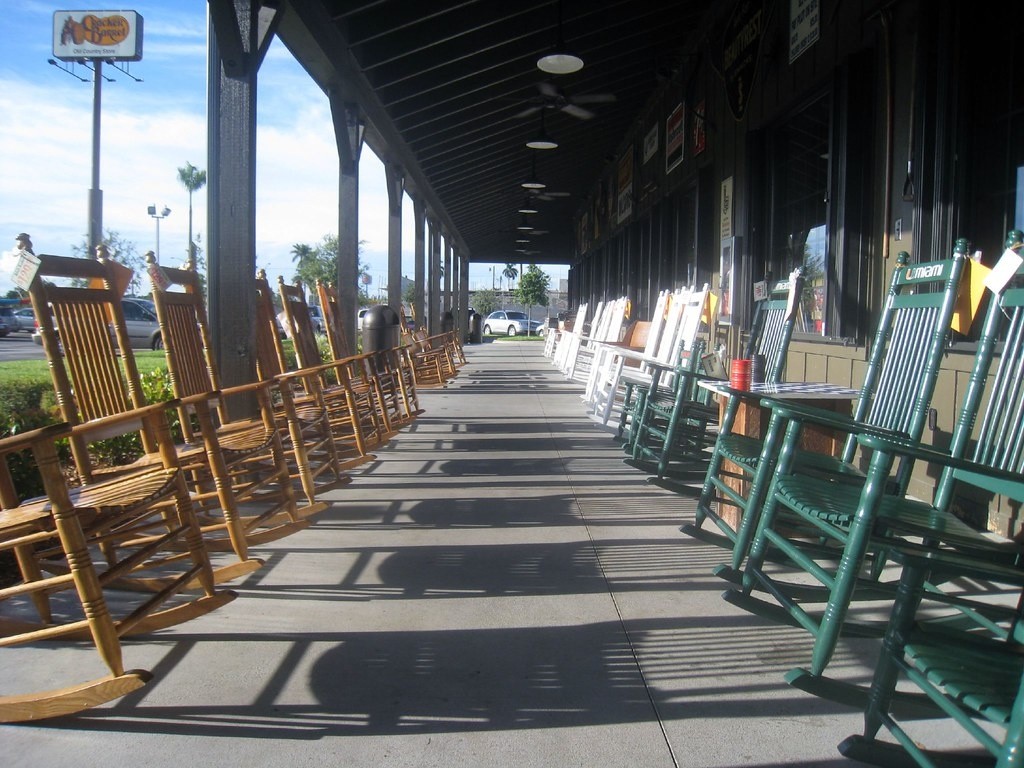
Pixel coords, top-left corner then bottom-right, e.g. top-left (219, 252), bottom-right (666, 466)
top-left (362, 305), bottom-right (401, 389)
top-left (470, 312), bottom-right (482, 343)
top-left (441, 312), bottom-right (454, 334)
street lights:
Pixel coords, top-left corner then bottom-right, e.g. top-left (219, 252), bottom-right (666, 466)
top-left (146, 205), bottom-right (172, 265)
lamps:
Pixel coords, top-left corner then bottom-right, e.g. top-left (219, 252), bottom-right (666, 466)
top-left (48, 55), bottom-right (144, 84)
top-left (513, 187), bottom-right (538, 254)
top-left (520, 149), bottom-right (546, 190)
top-left (536, 1), bottom-right (585, 76)
top-left (525, 101), bottom-right (560, 150)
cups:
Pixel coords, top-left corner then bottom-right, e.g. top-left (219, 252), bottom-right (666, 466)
top-left (748, 354), bottom-right (765, 383)
top-left (730, 359), bottom-right (751, 392)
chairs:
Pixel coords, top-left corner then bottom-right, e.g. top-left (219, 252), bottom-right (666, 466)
top-left (719, 229), bottom-right (1023, 768)
top-left (0, 398), bottom-right (239, 726)
top-left (540, 264), bottom-right (808, 484)
top-left (111, 249), bottom-right (470, 551)
top-left (677, 239), bottom-right (970, 601)
top-left (16, 231), bottom-right (310, 599)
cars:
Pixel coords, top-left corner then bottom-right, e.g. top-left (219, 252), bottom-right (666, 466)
top-left (403, 315), bottom-right (413, 324)
top-left (536, 324), bottom-right (545, 338)
top-left (311, 305), bottom-right (328, 339)
top-left (11, 307), bottom-right (40, 332)
top-left (481, 310), bottom-right (541, 337)
top-left (31, 297), bottom-right (165, 356)
top-left (0, 305), bottom-right (22, 337)
top-left (356, 308), bottom-right (369, 330)
